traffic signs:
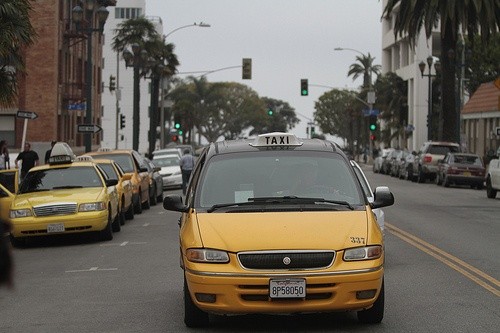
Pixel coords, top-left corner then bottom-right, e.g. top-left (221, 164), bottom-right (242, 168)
top-left (78, 125), bottom-right (102, 134)
top-left (17, 110), bottom-right (38, 120)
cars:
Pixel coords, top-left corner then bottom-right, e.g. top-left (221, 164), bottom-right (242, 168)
top-left (164, 138), bottom-right (395, 325)
top-left (145, 149), bottom-right (186, 189)
top-left (372, 147), bottom-right (413, 181)
top-left (349, 159), bottom-right (389, 234)
top-left (485, 146), bottom-right (500, 199)
top-left (0, 150), bottom-right (164, 249)
top-left (438, 152), bottom-right (486, 189)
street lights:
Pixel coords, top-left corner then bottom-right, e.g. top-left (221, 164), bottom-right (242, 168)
top-left (419, 56), bottom-right (442, 141)
top-left (73, 4), bottom-right (109, 152)
top-left (160, 23), bottom-right (211, 148)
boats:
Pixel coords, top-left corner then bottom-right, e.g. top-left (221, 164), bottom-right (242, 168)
top-left (268, 108), bottom-right (272, 115)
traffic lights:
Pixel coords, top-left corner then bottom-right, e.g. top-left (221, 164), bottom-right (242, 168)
top-left (120, 114), bottom-right (126, 130)
top-left (174, 111), bottom-right (183, 135)
top-left (110, 75), bottom-right (115, 91)
top-left (311, 127), bottom-right (315, 136)
top-left (370, 115), bottom-right (377, 130)
top-left (301, 79), bottom-right (308, 95)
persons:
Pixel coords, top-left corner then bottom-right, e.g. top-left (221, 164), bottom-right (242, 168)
top-left (44, 140), bottom-right (56, 165)
top-left (0, 140), bottom-right (9, 170)
top-left (15, 143), bottom-right (39, 184)
top-left (180, 149), bottom-right (196, 195)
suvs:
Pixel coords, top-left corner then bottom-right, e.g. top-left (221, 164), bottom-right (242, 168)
top-left (412, 141), bottom-right (461, 184)
